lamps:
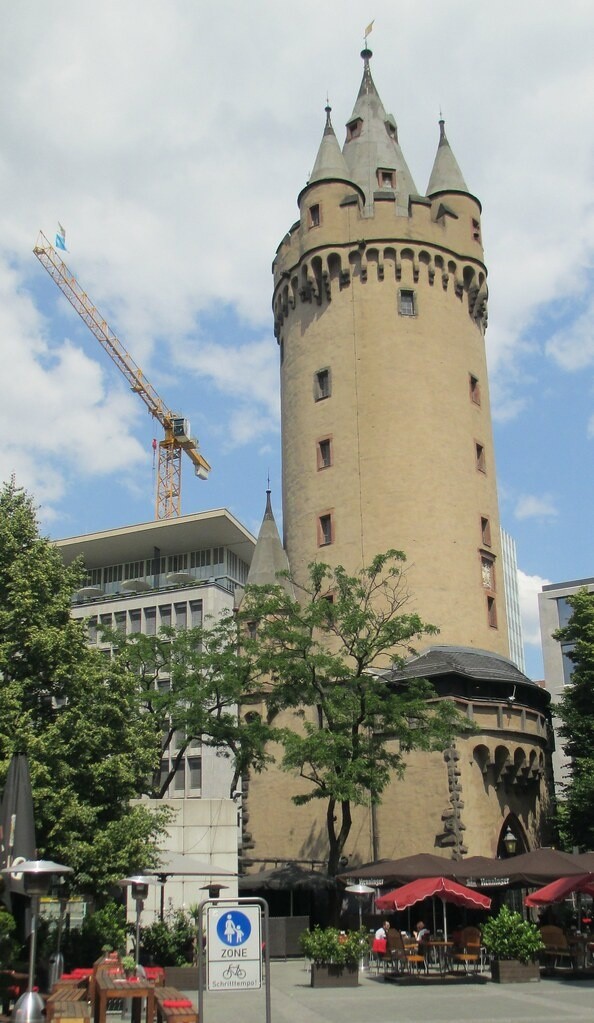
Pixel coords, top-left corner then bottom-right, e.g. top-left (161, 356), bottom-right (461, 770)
top-left (506, 684), bottom-right (517, 703)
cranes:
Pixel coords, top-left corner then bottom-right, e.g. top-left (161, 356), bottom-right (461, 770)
top-left (33, 229), bottom-right (212, 521)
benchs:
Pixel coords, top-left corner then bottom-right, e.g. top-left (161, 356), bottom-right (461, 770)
top-left (44, 966), bottom-right (199, 1023)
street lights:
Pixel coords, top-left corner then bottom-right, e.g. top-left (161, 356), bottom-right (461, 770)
top-left (502, 824), bottom-right (518, 915)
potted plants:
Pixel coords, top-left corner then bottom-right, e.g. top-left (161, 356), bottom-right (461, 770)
top-left (119, 904), bottom-right (207, 990)
top-left (101, 944), bottom-right (114, 959)
top-left (117, 949), bottom-right (136, 978)
top-left (480, 903), bottom-right (548, 984)
top-left (296, 924), bottom-right (370, 989)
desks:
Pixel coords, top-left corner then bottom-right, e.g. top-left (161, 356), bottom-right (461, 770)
top-left (425, 940), bottom-right (454, 982)
top-left (95, 966), bottom-right (156, 1023)
top-left (404, 942), bottom-right (417, 948)
top-left (566, 938), bottom-right (593, 969)
top-left (90, 954), bottom-right (139, 1018)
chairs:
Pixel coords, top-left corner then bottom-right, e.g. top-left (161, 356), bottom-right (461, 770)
top-left (366, 925), bottom-right (594, 982)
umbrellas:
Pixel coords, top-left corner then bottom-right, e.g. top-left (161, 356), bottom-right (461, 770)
top-left (144, 855), bottom-right (249, 920)
top-left (0, 751), bottom-right (42, 945)
top-left (238, 847), bottom-right (594, 969)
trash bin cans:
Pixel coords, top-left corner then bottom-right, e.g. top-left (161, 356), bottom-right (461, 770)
top-left (121, 964), bottom-right (148, 1020)
top-left (14, 990), bottom-right (46, 1022)
top-left (47, 952), bottom-right (66, 989)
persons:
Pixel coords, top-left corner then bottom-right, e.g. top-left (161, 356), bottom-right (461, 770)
top-left (375, 921), bottom-right (390, 968)
top-left (413, 922), bottom-right (428, 968)
